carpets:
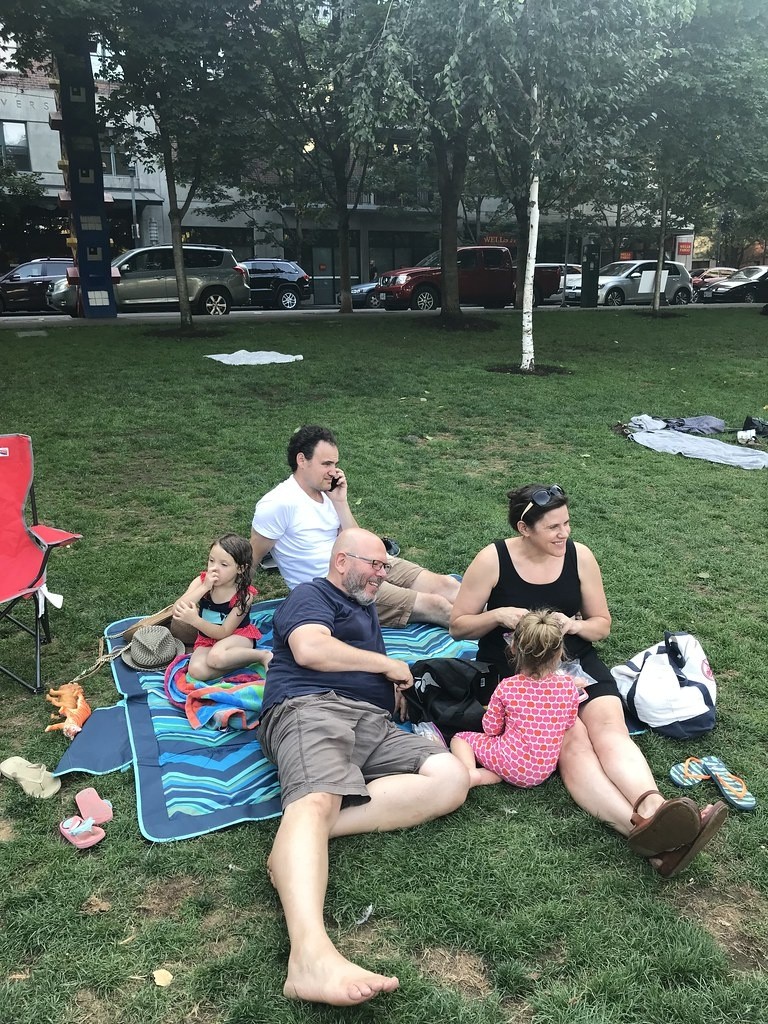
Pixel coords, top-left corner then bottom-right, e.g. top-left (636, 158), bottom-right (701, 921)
top-left (54, 573), bottom-right (480, 843)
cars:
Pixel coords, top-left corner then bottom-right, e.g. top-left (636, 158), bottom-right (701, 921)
top-left (510, 261), bottom-right (584, 307)
top-left (688, 265), bottom-right (741, 303)
top-left (698, 264), bottom-right (768, 304)
top-left (561, 258), bottom-right (693, 307)
top-left (338, 281), bottom-right (386, 309)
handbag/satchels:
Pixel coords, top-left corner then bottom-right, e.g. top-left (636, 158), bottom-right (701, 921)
top-left (611, 632), bottom-right (717, 738)
top-left (403, 659), bottom-right (493, 740)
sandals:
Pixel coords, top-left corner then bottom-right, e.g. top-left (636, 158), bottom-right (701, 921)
top-left (627, 791), bottom-right (700, 857)
top-left (647, 801), bottom-right (728, 879)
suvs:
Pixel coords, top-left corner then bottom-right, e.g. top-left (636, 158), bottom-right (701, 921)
top-left (238, 256), bottom-right (312, 310)
top-left (44, 240), bottom-right (253, 319)
top-left (0, 257), bottom-right (77, 316)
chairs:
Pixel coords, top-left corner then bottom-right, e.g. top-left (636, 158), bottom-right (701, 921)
top-left (0, 433), bottom-right (82, 693)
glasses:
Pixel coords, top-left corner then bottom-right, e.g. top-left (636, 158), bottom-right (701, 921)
top-left (521, 485), bottom-right (564, 521)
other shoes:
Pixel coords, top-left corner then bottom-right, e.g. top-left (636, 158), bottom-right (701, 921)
top-left (383, 538), bottom-right (401, 557)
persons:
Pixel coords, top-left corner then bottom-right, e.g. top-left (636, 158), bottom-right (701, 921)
top-left (369, 260), bottom-right (377, 280)
top-left (172, 533), bottom-right (274, 682)
top-left (255, 527), bottom-right (470, 1005)
top-left (450, 609), bottom-right (579, 788)
top-left (449, 485), bottom-right (728, 879)
top-left (250, 425), bottom-right (461, 629)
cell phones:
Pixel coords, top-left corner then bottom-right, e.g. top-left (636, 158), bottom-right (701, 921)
top-left (328, 477), bottom-right (340, 492)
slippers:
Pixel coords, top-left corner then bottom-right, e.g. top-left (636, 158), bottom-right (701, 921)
top-left (76, 788), bottom-right (113, 825)
top-left (671, 755), bottom-right (724, 788)
top-left (346, 554), bottom-right (391, 574)
top-left (0, 756), bottom-right (61, 798)
top-left (704, 760), bottom-right (756, 810)
top-left (59, 815), bottom-right (105, 849)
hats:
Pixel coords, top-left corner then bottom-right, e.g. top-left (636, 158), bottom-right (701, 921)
top-left (122, 626), bottom-right (185, 671)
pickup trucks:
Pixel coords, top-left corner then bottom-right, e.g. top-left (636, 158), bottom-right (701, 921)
top-left (375, 243), bottom-right (562, 311)
